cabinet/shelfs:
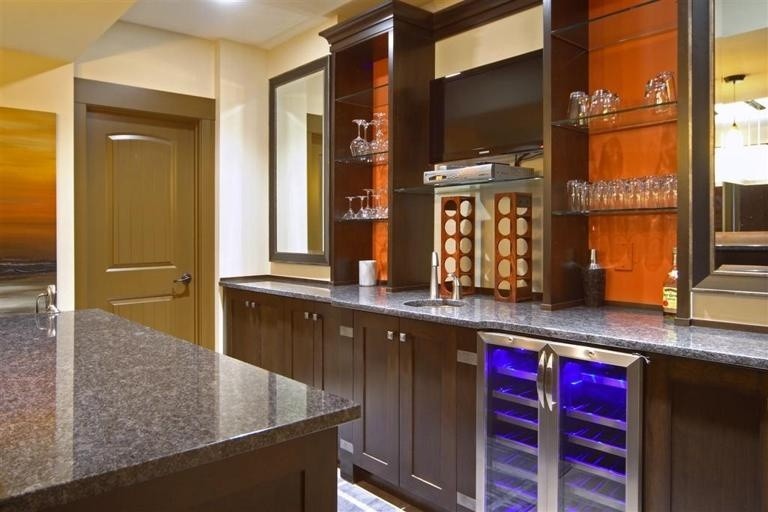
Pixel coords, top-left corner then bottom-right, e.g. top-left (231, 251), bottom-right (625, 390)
top-left (540, 0), bottom-right (711, 325)
top-left (318, 0), bottom-right (436, 292)
top-left (223, 286), bottom-right (768, 512)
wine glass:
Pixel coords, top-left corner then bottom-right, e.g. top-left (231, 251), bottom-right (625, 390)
top-left (342, 111), bottom-right (389, 219)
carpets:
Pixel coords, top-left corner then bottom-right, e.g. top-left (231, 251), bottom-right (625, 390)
top-left (337, 468), bottom-right (404, 512)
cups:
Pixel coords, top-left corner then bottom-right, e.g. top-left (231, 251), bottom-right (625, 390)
top-left (565, 175), bottom-right (677, 210)
top-left (569, 72), bottom-right (677, 127)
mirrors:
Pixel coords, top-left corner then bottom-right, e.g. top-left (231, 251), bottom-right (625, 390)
top-left (712, 27), bottom-right (768, 277)
top-left (268, 55), bottom-right (331, 266)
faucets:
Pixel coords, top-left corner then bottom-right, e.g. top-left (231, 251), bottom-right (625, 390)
top-left (430, 252), bottom-right (439, 299)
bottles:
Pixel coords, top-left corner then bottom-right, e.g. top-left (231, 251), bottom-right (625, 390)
top-left (582, 248), bottom-right (604, 307)
top-left (662, 246), bottom-right (678, 314)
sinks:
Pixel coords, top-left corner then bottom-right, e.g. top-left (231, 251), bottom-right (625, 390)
top-left (403, 299), bottom-right (470, 308)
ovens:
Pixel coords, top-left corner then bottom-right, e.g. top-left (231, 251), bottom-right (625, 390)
top-left (474, 331), bottom-right (644, 512)
